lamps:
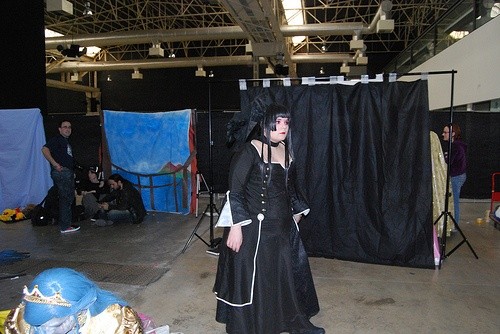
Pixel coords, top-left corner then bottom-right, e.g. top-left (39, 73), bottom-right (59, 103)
top-left (349, 33), bottom-right (364, 50)
top-left (132, 70), bottom-right (143, 79)
top-left (46, 0), bottom-right (73, 15)
top-left (356, 52), bottom-right (368, 65)
top-left (56, 43), bottom-right (88, 57)
top-left (149, 42), bottom-right (164, 57)
top-left (83, 3), bottom-right (93, 15)
top-left (340, 61), bottom-right (350, 73)
top-left (376, 12), bottom-right (394, 33)
top-left (265, 64), bottom-right (274, 74)
top-left (169, 49), bottom-right (175, 58)
top-left (322, 41), bottom-right (328, 51)
top-left (196, 66), bottom-right (206, 77)
top-left (245, 40), bottom-right (252, 53)
top-left (70, 71), bottom-right (82, 82)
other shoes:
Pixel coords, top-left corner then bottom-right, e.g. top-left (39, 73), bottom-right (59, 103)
top-left (59, 225), bottom-right (80, 233)
top-left (94, 220), bottom-right (113, 227)
top-left (90, 211), bottom-right (104, 221)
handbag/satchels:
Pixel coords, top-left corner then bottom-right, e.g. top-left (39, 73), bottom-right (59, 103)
top-left (31, 203), bottom-right (51, 227)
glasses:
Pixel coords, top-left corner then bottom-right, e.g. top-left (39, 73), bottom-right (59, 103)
top-left (443, 131), bottom-right (449, 134)
top-left (60, 127), bottom-right (71, 129)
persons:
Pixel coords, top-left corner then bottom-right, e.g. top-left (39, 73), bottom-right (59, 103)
top-left (95, 174), bottom-right (146, 227)
top-left (82, 169), bottom-right (108, 218)
top-left (43, 121), bottom-right (81, 233)
top-left (213, 103), bottom-right (325, 334)
top-left (5, 268), bottom-right (169, 334)
top-left (443, 122), bottom-right (466, 223)
